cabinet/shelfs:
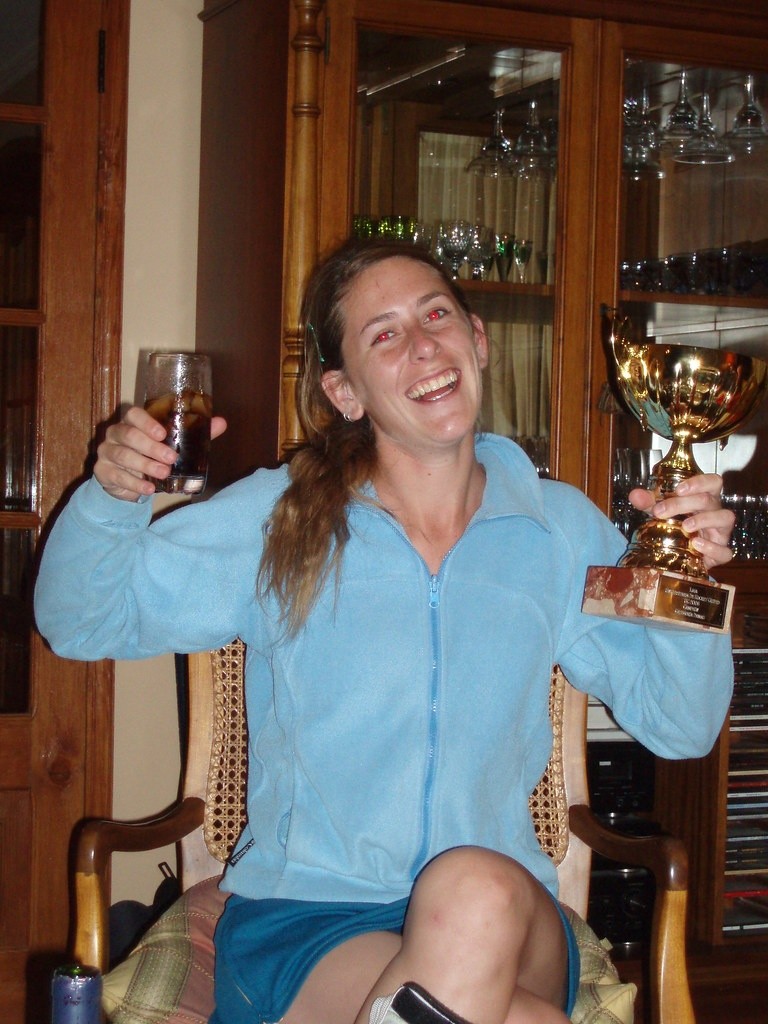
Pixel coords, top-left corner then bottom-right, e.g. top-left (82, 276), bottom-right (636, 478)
top-left (197, 0), bottom-right (768, 535)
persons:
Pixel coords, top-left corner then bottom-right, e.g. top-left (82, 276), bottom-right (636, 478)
top-left (33, 234), bottom-right (736, 1024)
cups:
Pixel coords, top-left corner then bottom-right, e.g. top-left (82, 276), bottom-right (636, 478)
top-left (624, 77), bottom-right (650, 129)
top-left (354, 216), bottom-right (432, 250)
top-left (143, 353), bottom-right (211, 495)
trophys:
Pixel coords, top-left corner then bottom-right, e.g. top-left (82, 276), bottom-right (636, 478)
top-left (581, 316), bottom-right (768, 634)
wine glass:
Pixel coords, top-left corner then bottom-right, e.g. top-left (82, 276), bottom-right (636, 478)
top-left (732, 74), bottom-right (768, 153)
top-left (664, 64), bottom-right (697, 140)
top-left (613, 472), bottom-right (768, 560)
top-left (671, 67), bottom-right (736, 164)
top-left (621, 57), bottom-right (669, 181)
top-left (437, 221), bottom-right (532, 284)
top-left (618, 247), bottom-right (768, 296)
top-left (468, 100), bottom-right (558, 180)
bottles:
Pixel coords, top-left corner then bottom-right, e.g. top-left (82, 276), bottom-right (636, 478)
top-left (51, 965), bottom-right (102, 1024)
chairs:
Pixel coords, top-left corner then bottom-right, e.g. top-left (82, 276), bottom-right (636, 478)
top-left (74, 637), bottom-right (695, 1024)
